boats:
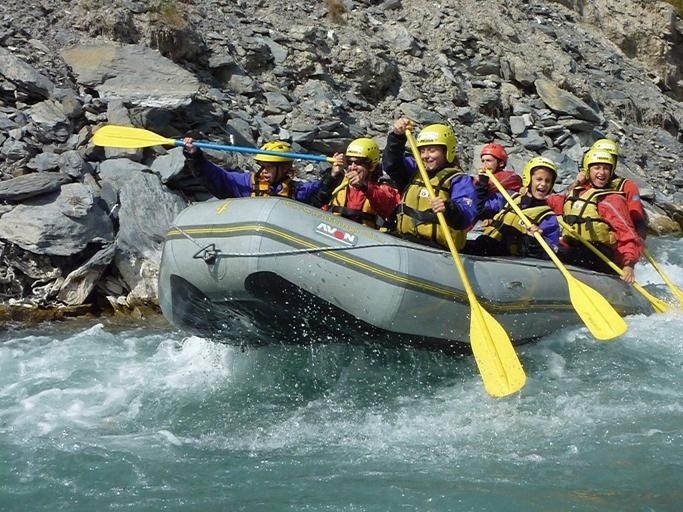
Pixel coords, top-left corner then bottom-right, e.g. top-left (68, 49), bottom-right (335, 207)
top-left (158, 198), bottom-right (670, 353)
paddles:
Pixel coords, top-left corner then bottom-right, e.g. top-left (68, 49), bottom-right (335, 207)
top-left (643, 249), bottom-right (683, 304)
top-left (555, 216), bottom-right (673, 316)
top-left (402, 119), bottom-right (526, 398)
top-left (92, 125), bottom-right (337, 164)
top-left (485, 170), bottom-right (627, 339)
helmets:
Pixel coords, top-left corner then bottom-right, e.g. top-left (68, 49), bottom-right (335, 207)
top-left (590, 138), bottom-right (619, 170)
top-left (521, 157), bottom-right (558, 196)
top-left (345, 137), bottom-right (380, 168)
top-left (583, 148), bottom-right (615, 179)
top-left (252, 141), bottom-right (296, 164)
top-left (414, 124), bottom-right (457, 164)
top-left (479, 143), bottom-right (508, 166)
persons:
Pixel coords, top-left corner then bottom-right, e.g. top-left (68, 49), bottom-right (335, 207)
top-left (466, 156), bottom-right (561, 261)
top-left (565, 136), bottom-right (650, 242)
top-left (379, 117), bottom-right (477, 252)
top-left (544, 148), bottom-right (646, 286)
top-left (181, 134), bottom-right (345, 208)
top-left (320, 136), bottom-right (400, 228)
top-left (462, 143), bottom-right (524, 256)
